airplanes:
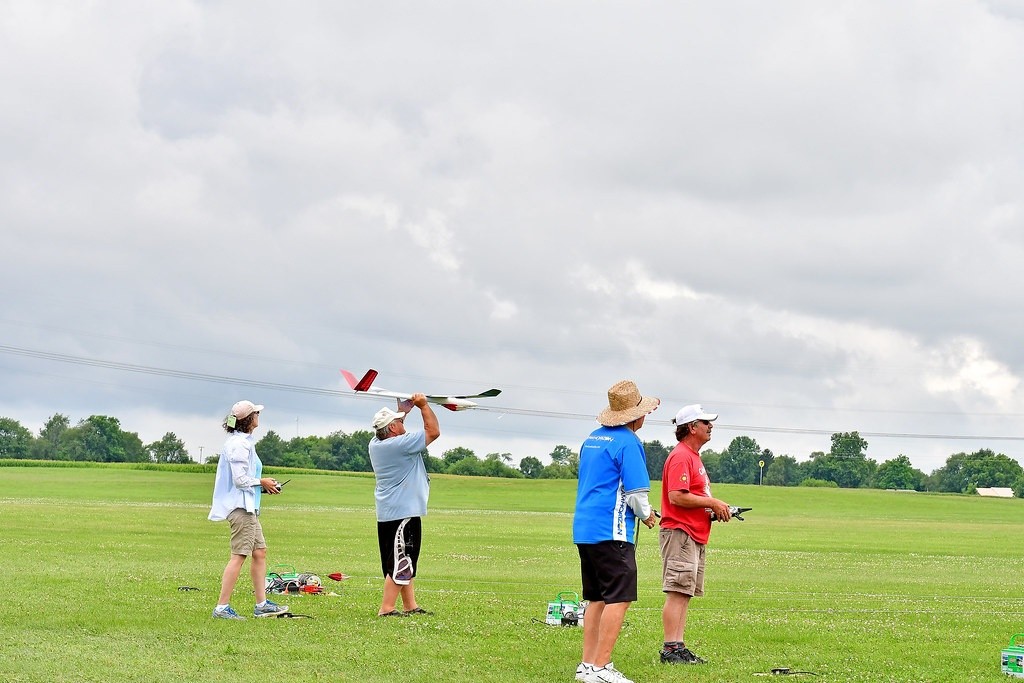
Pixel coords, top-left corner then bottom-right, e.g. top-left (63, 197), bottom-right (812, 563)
top-left (343, 367), bottom-right (501, 412)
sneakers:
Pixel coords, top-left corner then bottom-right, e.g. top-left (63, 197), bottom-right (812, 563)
top-left (678, 647), bottom-right (707, 665)
top-left (583, 662), bottom-right (634, 683)
top-left (253, 600), bottom-right (290, 617)
top-left (213, 605), bottom-right (242, 620)
top-left (575, 662), bottom-right (590, 680)
top-left (659, 647), bottom-right (688, 665)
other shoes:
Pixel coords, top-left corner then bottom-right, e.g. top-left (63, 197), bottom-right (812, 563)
top-left (381, 609), bottom-right (403, 616)
top-left (405, 607), bottom-right (433, 615)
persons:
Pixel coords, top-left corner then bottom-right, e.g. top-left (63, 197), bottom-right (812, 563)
top-left (571, 381), bottom-right (661, 683)
top-left (207, 400), bottom-right (289, 619)
top-left (658, 404), bottom-right (731, 665)
top-left (368, 393), bottom-right (441, 617)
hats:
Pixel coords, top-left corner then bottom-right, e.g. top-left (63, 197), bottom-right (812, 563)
top-left (372, 407), bottom-right (405, 430)
top-left (596, 380), bottom-right (661, 427)
top-left (230, 401), bottom-right (264, 420)
top-left (670, 404), bottom-right (718, 427)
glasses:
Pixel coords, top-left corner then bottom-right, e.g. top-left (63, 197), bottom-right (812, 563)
top-left (254, 411), bottom-right (260, 414)
top-left (394, 418), bottom-right (403, 424)
top-left (692, 420), bottom-right (709, 426)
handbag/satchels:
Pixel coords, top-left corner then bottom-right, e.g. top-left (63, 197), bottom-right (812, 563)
top-left (545, 591), bottom-right (585, 629)
